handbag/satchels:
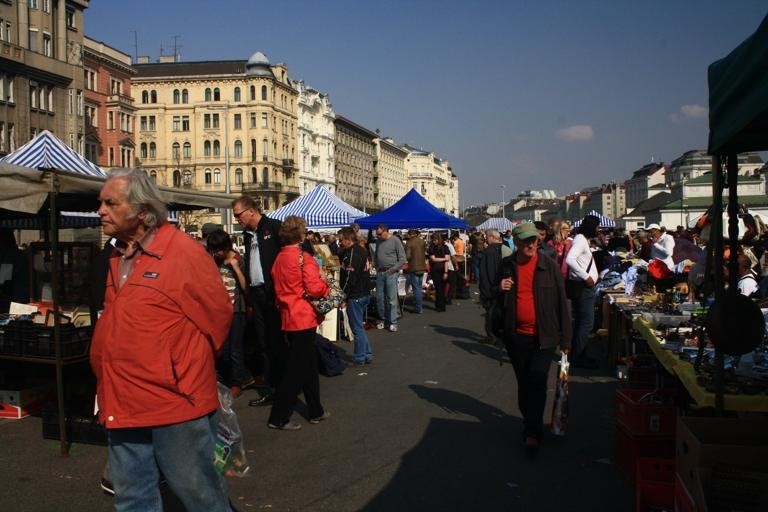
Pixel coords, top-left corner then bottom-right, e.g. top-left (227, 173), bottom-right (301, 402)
top-left (549, 351), bottom-right (570, 438)
top-left (458, 284), bottom-right (470, 299)
top-left (562, 274), bottom-right (583, 300)
top-left (301, 269), bottom-right (347, 314)
top-left (313, 332), bottom-right (342, 378)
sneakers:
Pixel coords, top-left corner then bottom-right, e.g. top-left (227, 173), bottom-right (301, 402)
top-left (375, 321), bottom-right (388, 329)
top-left (229, 386), bottom-right (241, 398)
top-left (309, 410), bottom-right (331, 424)
top-left (364, 357), bottom-right (373, 364)
top-left (100, 476), bottom-right (114, 495)
top-left (267, 420), bottom-right (301, 432)
top-left (240, 377), bottom-right (255, 389)
top-left (388, 323), bottom-right (398, 332)
top-left (444, 297), bottom-right (451, 304)
top-left (346, 362), bottom-right (364, 368)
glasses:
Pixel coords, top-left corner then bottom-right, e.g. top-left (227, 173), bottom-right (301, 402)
top-left (375, 229), bottom-right (383, 235)
top-left (234, 206), bottom-right (249, 218)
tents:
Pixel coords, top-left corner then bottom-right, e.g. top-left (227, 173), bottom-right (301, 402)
top-left (267, 184), bottom-right (472, 274)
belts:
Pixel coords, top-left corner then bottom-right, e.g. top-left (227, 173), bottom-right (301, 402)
top-left (378, 267), bottom-right (398, 272)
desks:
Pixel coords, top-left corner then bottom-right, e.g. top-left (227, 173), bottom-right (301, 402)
top-left (600, 282), bottom-right (768, 506)
top-left (1, 355), bottom-right (103, 457)
top-left (365, 273), bottom-right (428, 323)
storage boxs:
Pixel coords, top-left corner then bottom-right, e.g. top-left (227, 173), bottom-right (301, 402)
top-left (637, 457), bottom-right (675, 512)
top-left (615, 388), bottom-right (676, 440)
top-left (0, 381), bottom-right (46, 419)
top-left (676, 416), bottom-right (767, 511)
top-left (615, 427), bottom-right (676, 482)
top-left (65, 367), bottom-right (96, 417)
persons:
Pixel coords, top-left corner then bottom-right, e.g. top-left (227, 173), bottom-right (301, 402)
top-left (101, 430), bottom-right (167, 496)
top-left (203, 195), bottom-right (768, 451)
top-left (89, 169), bottom-right (235, 512)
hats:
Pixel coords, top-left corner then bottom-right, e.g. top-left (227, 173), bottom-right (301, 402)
top-left (511, 222), bottom-right (539, 241)
top-left (632, 231), bottom-right (645, 240)
top-left (643, 224), bottom-right (661, 231)
top-left (201, 223), bottom-right (220, 234)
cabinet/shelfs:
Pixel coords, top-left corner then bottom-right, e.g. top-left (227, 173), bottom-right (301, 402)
top-left (28, 241), bottom-right (102, 308)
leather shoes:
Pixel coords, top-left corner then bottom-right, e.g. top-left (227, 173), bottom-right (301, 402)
top-left (249, 393), bottom-right (273, 407)
